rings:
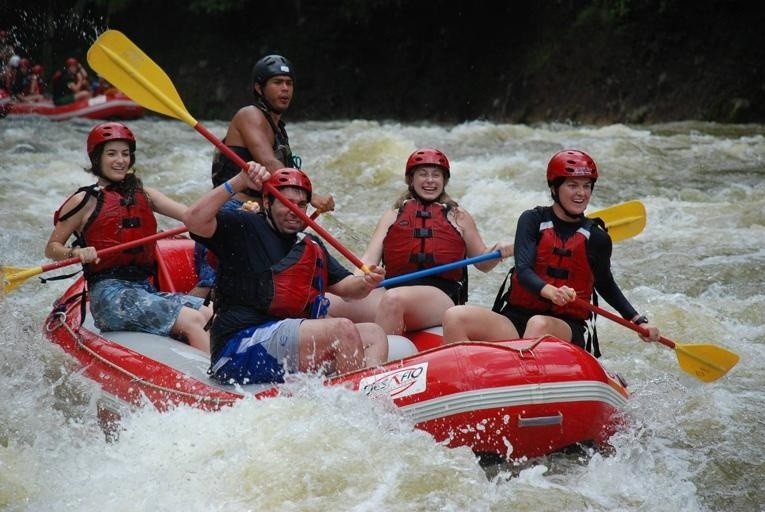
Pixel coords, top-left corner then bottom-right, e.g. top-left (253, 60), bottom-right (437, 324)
top-left (559, 298), bottom-right (565, 302)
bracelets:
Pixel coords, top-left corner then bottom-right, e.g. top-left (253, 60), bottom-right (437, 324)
top-left (223, 179), bottom-right (236, 198)
top-left (68, 244), bottom-right (78, 258)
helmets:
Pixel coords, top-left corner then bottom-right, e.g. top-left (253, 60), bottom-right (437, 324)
top-left (545, 149), bottom-right (600, 188)
top-left (404, 147), bottom-right (451, 177)
top-left (66, 57), bottom-right (80, 65)
top-left (32, 66), bottom-right (45, 73)
top-left (19, 58), bottom-right (32, 66)
top-left (86, 122), bottom-right (138, 158)
top-left (250, 54), bottom-right (295, 85)
top-left (265, 168), bottom-right (313, 202)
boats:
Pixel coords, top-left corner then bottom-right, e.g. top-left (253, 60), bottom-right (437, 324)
top-left (0, 88), bottom-right (143, 120)
top-left (42, 237), bottom-right (627, 468)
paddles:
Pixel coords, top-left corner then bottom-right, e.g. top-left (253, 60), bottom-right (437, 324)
top-left (0, 225), bottom-right (191, 297)
top-left (85, 30), bottom-right (372, 286)
top-left (373, 199), bottom-right (647, 288)
top-left (569, 294), bottom-right (740, 384)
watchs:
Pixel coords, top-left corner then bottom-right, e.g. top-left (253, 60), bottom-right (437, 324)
top-left (632, 316), bottom-right (649, 327)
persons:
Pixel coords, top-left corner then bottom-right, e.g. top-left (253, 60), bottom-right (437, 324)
top-left (212, 54), bottom-right (335, 214)
top-left (442, 148), bottom-right (661, 346)
top-left (44, 122), bottom-right (217, 353)
top-left (182, 162), bottom-right (389, 386)
top-left (320, 147), bottom-right (515, 336)
top-left (0, 29), bottom-right (115, 106)
